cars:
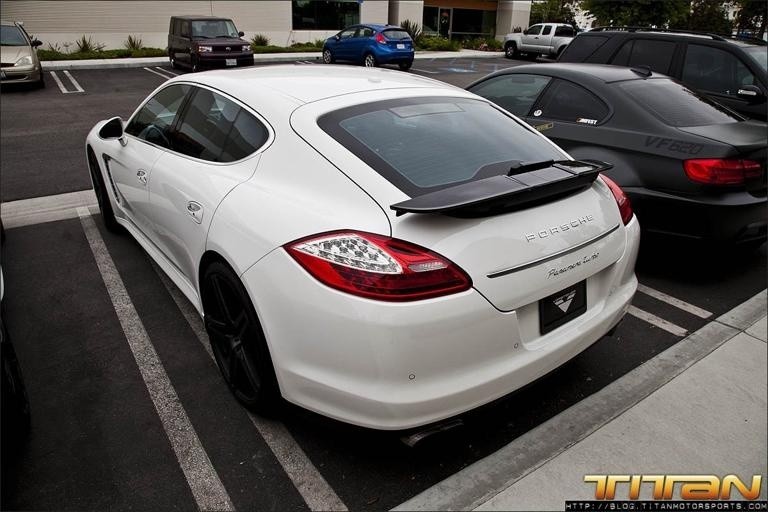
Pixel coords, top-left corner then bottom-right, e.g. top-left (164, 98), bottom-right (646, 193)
top-left (0, 22), bottom-right (42, 83)
